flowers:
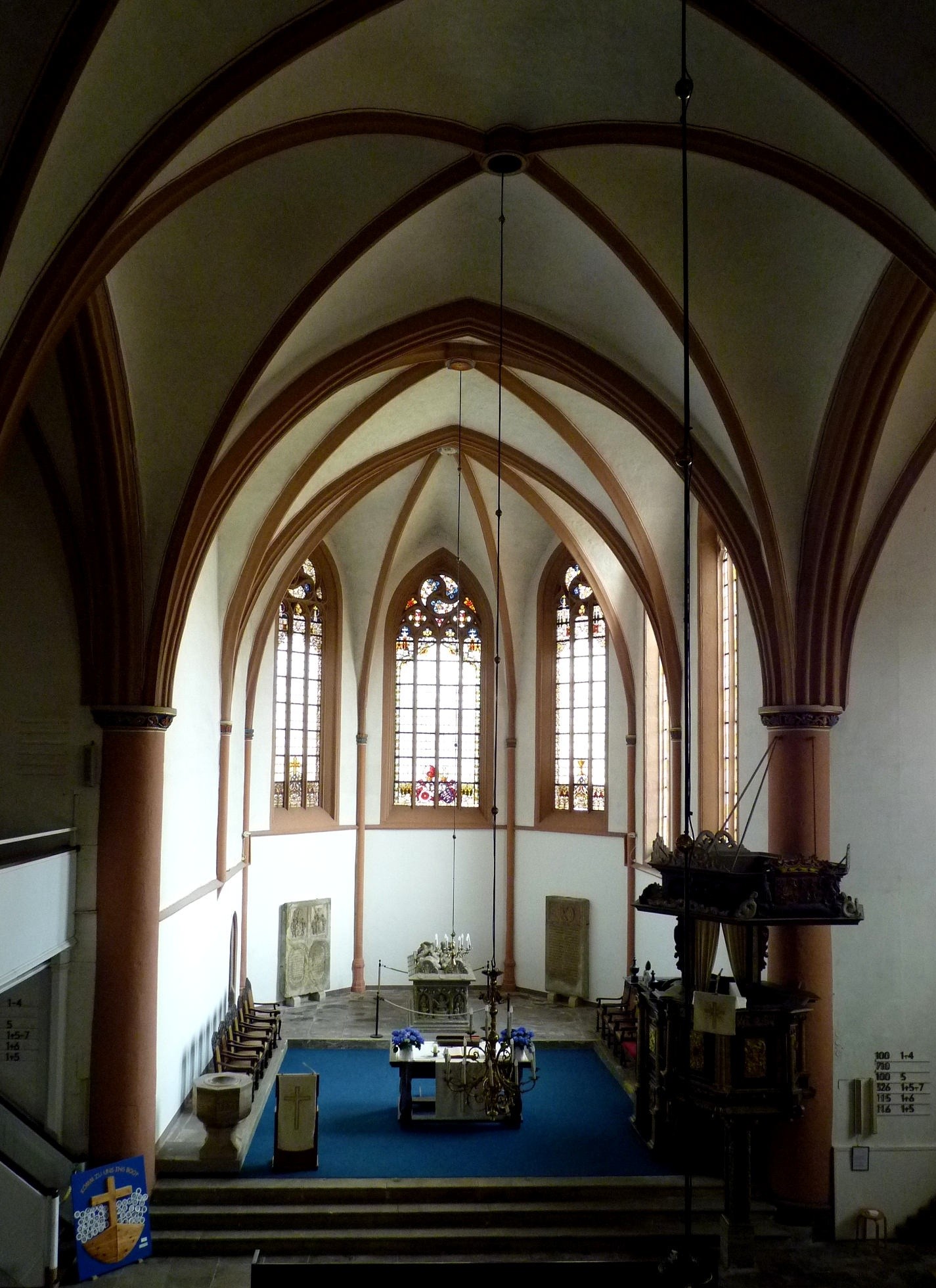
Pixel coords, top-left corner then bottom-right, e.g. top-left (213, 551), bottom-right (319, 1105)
top-left (392, 1026), bottom-right (424, 1053)
top-left (498, 1027), bottom-right (535, 1056)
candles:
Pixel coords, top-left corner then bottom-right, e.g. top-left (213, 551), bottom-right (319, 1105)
top-left (434, 1043), bottom-right (438, 1053)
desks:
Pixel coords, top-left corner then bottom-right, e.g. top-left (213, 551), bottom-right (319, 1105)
top-left (390, 1041), bottom-right (532, 1128)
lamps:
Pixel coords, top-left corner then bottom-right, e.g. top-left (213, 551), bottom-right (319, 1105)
top-left (443, 125), bottom-right (539, 1121)
top-left (435, 357), bottom-right (473, 964)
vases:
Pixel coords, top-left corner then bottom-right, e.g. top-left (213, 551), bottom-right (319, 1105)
top-left (515, 1047), bottom-right (523, 1056)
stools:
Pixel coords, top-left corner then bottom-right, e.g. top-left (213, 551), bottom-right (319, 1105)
top-left (856, 1209), bottom-right (887, 1252)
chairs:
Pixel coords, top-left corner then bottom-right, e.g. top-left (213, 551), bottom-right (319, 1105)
top-left (596, 981), bottom-right (646, 1069)
top-left (211, 978), bottom-right (281, 1103)
